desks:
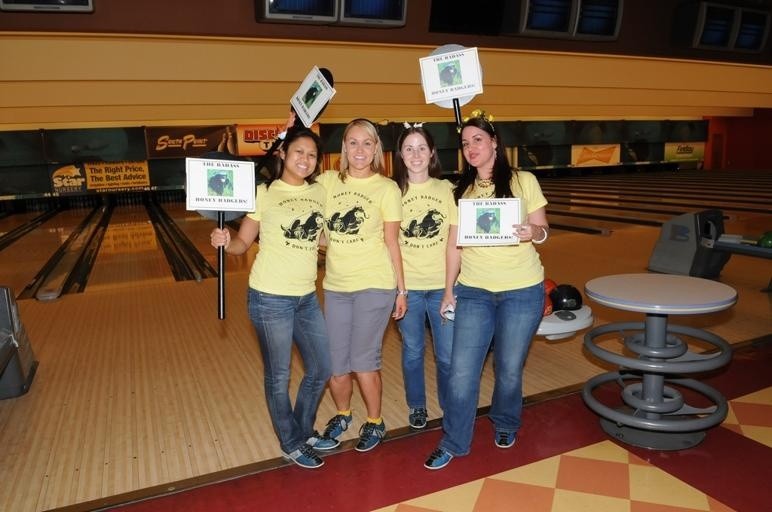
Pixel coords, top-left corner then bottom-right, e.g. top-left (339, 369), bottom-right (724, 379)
top-left (582, 273), bottom-right (738, 454)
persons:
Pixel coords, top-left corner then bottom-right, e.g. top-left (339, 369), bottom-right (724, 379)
top-left (209, 124), bottom-right (343, 469)
top-left (387, 120), bottom-right (465, 428)
top-left (421, 106), bottom-right (552, 470)
top-left (313, 115), bottom-right (410, 453)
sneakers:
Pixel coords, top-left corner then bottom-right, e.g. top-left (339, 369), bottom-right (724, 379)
top-left (280, 411), bottom-right (519, 469)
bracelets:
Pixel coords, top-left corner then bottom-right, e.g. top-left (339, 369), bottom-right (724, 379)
top-left (531, 227), bottom-right (547, 245)
top-left (396, 289), bottom-right (408, 296)
